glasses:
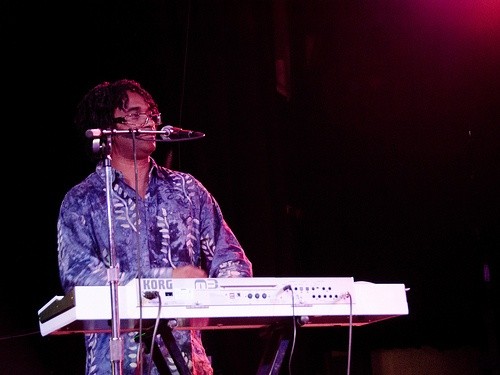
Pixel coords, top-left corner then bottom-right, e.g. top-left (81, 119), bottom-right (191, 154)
top-left (111, 112), bottom-right (161, 127)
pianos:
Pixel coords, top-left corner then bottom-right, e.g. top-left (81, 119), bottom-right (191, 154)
top-left (37, 277), bottom-right (409, 339)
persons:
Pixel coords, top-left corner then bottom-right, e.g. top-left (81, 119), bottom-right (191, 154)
top-left (56, 80), bottom-right (253, 375)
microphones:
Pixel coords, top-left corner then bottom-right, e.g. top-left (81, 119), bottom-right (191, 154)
top-left (160, 125), bottom-right (205, 138)
